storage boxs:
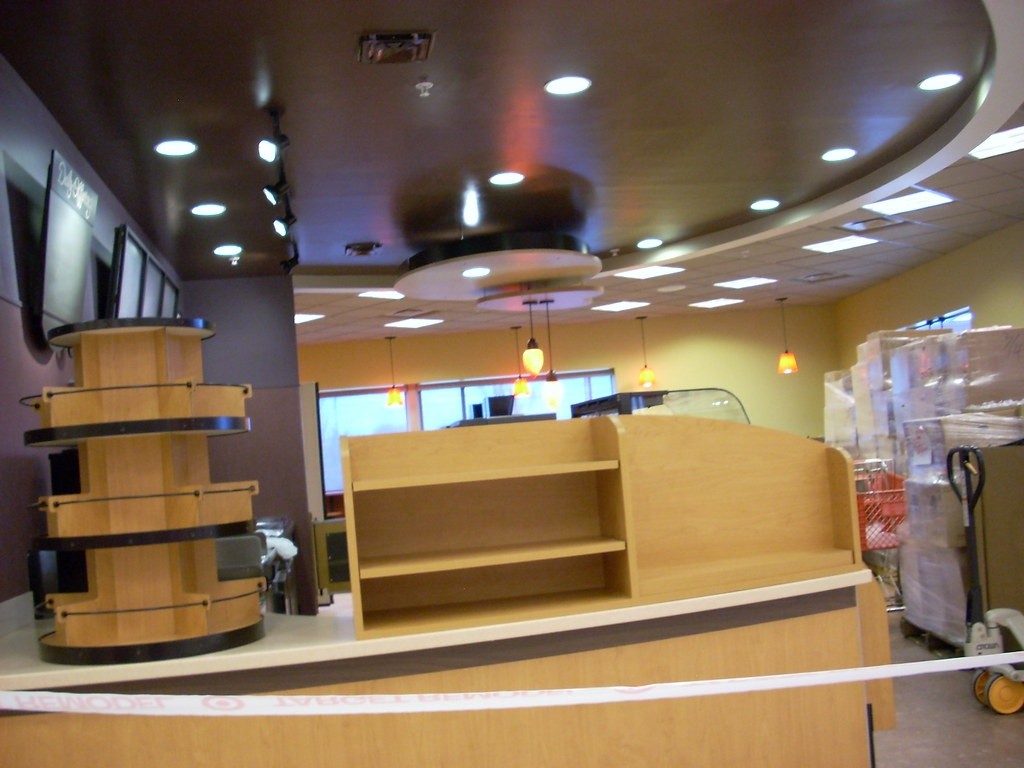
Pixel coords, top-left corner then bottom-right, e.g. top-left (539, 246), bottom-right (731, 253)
top-left (822, 324), bottom-right (1024, 648)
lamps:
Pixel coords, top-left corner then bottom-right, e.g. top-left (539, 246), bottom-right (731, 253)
top-left (635, 316), bottom-right (656, 387)
top-left (384, 336), bottom-right (403, 407)
top-left (509, 298), bottom-right (561, 399)
top-left (775, 298), bottom-right (798, 375)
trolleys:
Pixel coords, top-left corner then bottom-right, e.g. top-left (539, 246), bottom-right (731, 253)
top-left (851, 459), bottom-right (907, 611)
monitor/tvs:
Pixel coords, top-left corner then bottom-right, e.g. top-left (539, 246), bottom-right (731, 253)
top-left (105, 224), bottom-right (180, 320)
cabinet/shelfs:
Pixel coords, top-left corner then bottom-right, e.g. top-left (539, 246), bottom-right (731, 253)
top-left (340, 414), bottom-right (641, 641)
top-left (312, 517), bottom-right (351, 595)
top-left (21, 315), bottom-right (274, 665)
